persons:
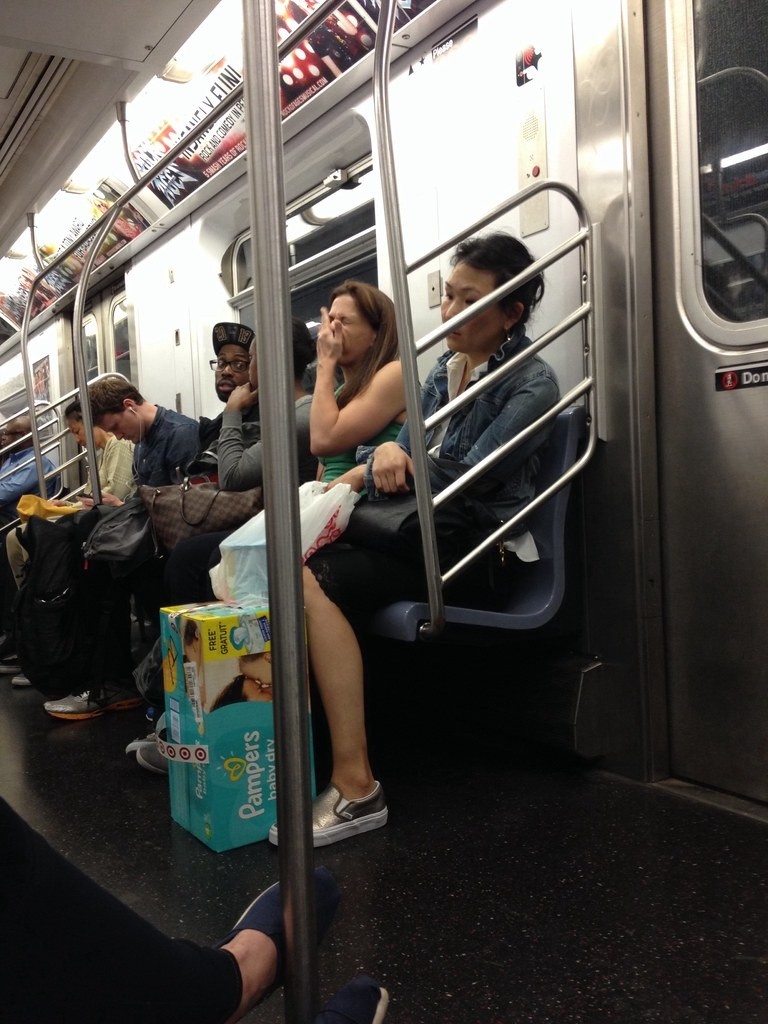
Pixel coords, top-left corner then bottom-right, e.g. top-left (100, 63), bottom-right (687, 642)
top-left (44, 380), bottom-right (203, 720)
top-left (1, 400), bottom-right (135, 685)
top-left (269, 235), bottom-right (560, 846)
top-left (312, 279), bottom-right (419, 481)
top-left (0, 415), bottom-right (61, 645)
top-left (146, 323), bottom-right (262, 721)
top-left (124, 316), bottom-right (317, 774)
top-left (0, 795), bottom-right (389, 1024)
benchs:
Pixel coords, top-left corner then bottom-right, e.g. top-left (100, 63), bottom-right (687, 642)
top-left (350, 404), bottom-right (595, 641)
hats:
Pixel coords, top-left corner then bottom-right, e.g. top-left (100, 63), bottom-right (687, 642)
top-left (212, 322), bottom-right (254, 355)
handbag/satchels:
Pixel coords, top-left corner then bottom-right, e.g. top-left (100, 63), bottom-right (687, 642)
top-left (79, 499), bottom-right (160, 568)
top-left (139, 477), bottom-right (264, 552)
top-left (343, 489), bottom-right (503, 556)
top-left (209, 480), bottom-right (361, 611)
top-left (17, 493), bottom-right (82, 522)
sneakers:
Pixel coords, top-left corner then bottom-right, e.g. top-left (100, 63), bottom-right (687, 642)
top-left (43, 679), bottom-right (143, 720)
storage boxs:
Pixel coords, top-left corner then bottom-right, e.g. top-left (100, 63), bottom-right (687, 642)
top-left (160, 599), bottom-right (317, 856)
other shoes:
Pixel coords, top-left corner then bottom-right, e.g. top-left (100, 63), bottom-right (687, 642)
top-left (0, 634), bottom-right (33, 687)
top-left (146, 707), bottom-right (154, 722)
top-left (125, 732), bottom-right (167, 775)
top-left (268, 780), bottom-right (388, 849)
top-left (317, 974), bottom-right (388, 1024)
top-left (210, 864), bottom-right (340, 1024)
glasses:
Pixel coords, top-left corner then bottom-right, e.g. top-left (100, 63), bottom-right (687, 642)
top-left (2, 429), bottom-right (20, 435)
top-left (209, 360), bottom-right (249, 373)
